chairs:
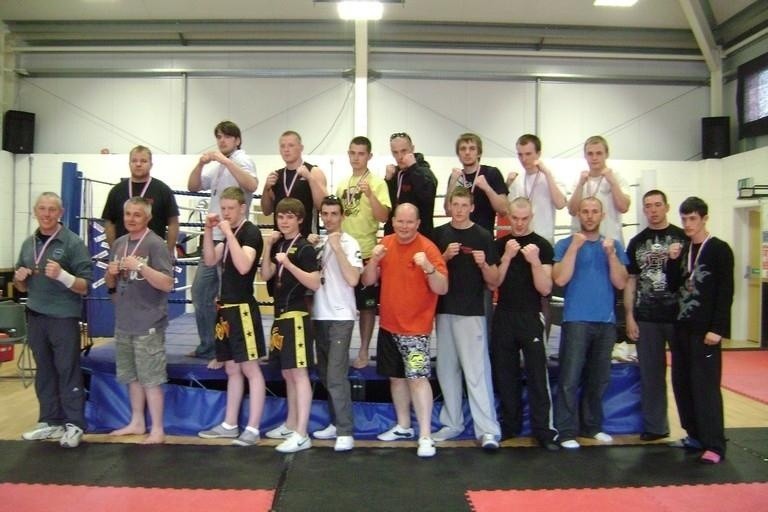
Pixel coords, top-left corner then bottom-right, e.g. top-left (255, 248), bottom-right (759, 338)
top-left (0, 302), bottom-right (33, 377)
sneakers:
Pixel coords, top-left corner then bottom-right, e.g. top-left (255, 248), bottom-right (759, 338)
top-left (333, 434), bottom-right (354, 452)
top-left (265, 422), bottom-right (295, 439)
top-left (376, 423), bottom-right (415, 442)
top-left (481, 432), bottom-right (500, 449)
top-left (416, 436), bottom-right (437, 458)
top-left (274, 431), bottom-right (313, 454)
top-left (430, 426), bottom-right (461, 442)
top-left (59, 422), bottom-right (85, 449)
top-left (231, 429), bottom-right (261, 447)
top-left (197, 424), bottom-right (240, 439)
top-left (312, 422), bottom-right (337, 440)
top-left (21, 422), bottom-right (66, 441)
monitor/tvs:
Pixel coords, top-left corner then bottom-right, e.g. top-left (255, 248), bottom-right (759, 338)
top-left (736, 51), bottom-right (768, 141)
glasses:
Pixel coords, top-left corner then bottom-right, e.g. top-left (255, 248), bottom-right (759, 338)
top-left (323, 195), bottom-right (340, 202)
top-left (390, 132), bottom-right (407, 140)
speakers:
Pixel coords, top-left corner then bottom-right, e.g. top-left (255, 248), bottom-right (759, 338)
top-left (3, 109), bottom-right (36, 154)
top-left (701, 116), bottom-right (730, 159)
